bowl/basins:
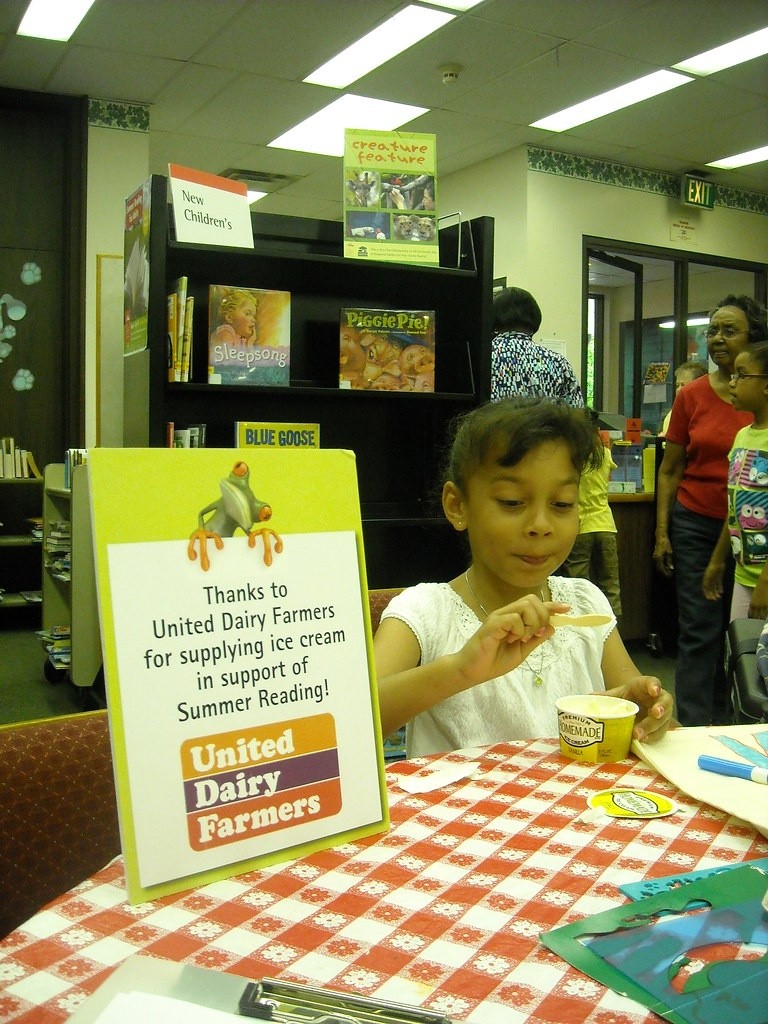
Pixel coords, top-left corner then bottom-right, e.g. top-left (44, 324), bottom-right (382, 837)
top-left (554, 694), bottom-right (640, 763)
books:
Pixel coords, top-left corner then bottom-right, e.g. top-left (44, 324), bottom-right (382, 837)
top-left (20, 590), bottom-right (42, 601)
top-left (0, 436), bottom-right (42, 479)
top-left (167, 422), bottom-right (208, 448)
top-left (25, 517), bottom-right (43, 542)
top-left (168, 276), bottom-right (194, 384)
top-left (34, 625), bottom-right (71, 670)
top-left (44, 520), bottom-right (71, 583)
top-left (65, 449), bottom-right (87, 489)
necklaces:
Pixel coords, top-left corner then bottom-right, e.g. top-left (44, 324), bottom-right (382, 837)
top-left (466, 567), bottom-right (545, 688)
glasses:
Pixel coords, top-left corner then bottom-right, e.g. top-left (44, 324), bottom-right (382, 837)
top-left (703, 328), bottom-right (757, 338)
top-left (730, 372), bottom-right (768, 380)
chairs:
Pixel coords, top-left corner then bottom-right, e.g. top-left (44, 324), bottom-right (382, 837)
top-left (0, 709), bottom-right (121, 940)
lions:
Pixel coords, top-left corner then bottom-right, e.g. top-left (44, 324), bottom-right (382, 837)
top-left (392, 215), bottom-right (436, 242)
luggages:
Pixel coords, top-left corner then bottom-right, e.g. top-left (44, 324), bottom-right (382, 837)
top-left (723, 617), bottom-right (768, 725)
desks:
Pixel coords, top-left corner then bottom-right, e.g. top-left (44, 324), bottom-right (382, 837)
top-left (0, 737), bottom-right (768, 1024)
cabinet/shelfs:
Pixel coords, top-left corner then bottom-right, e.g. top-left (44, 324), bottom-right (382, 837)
top-left (123, 173), bottom-right (495, 588)
top-left (0, 475), bottom-right (42, 607)
top-left (44, 464), bottom-right (101, 689)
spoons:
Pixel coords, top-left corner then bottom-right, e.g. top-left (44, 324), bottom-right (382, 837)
top-left (548, 614), bottom-right (612, 628)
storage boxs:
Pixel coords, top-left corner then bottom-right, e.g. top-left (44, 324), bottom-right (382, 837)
top-left (609, 481), bottom-right (636, 494)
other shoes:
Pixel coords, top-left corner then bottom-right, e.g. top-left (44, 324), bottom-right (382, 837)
top-left (648, 632), bottom-right (664, 658)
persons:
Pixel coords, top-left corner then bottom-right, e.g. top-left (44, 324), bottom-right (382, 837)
top-left (702, 340), bottom-right (768, 623)
top-left (422, 183), bottom-right (436, 211)
top-left (491, 287), bottom-right (581, 408)
top-left (374, 394), bottom-right (675, 745)
top-left (653, 294), bottom-right (768, 727)
top-left (564, 414), bottom-right (622, 614)
top-left (662, 360), bottom-right (709, 436)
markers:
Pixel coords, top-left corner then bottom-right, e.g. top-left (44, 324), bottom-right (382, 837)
top-left (698, 755), bottom-right (766, 783)
